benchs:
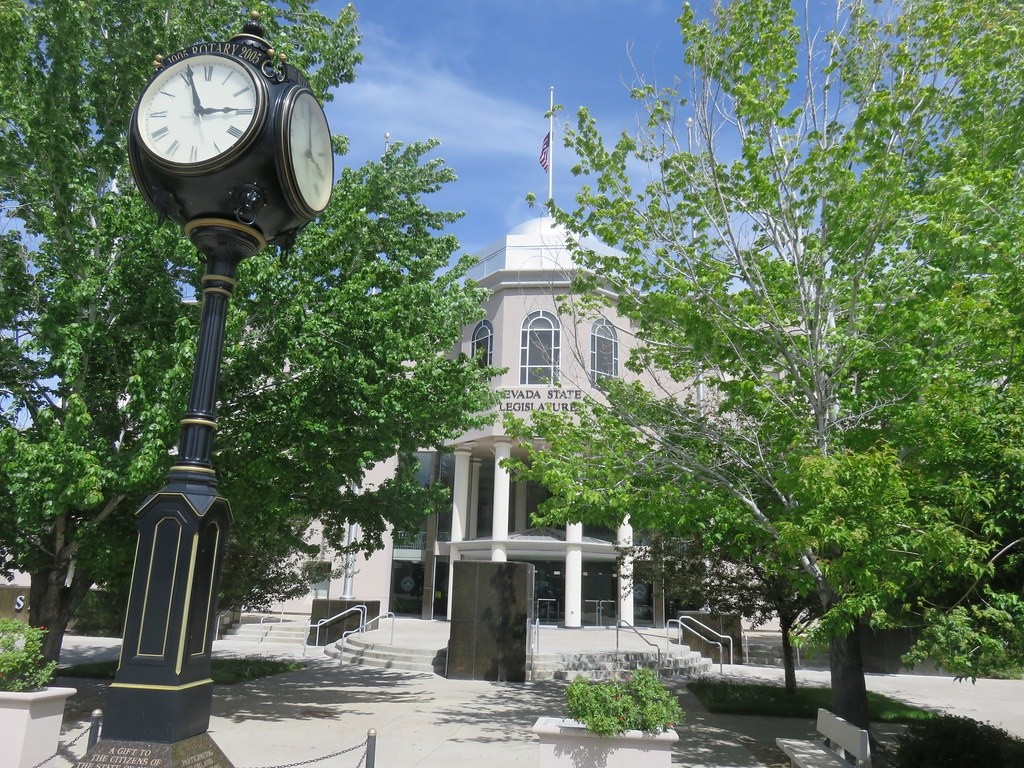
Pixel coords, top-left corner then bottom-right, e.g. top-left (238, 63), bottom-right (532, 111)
top-left (775, 707), bottom-right (873, 768)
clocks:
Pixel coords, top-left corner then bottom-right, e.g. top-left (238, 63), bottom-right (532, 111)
top-left (273, 84), bottom-right (334, 221)
top-left (135, 54), bottom-right (270, 178)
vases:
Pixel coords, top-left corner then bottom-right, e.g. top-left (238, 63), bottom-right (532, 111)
top-left (532, 716), bottom-right (680, 768)
top-left (0, 686), bottom-right (78, 768)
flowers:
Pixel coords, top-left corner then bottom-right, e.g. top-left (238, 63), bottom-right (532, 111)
top-left (0, 616), bottom-right (59, 693)
top-left (561, 667), bottom-right (688, 740)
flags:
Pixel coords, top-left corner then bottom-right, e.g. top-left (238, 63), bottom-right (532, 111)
top-left (540, 132), bottom-right (551, 173)
